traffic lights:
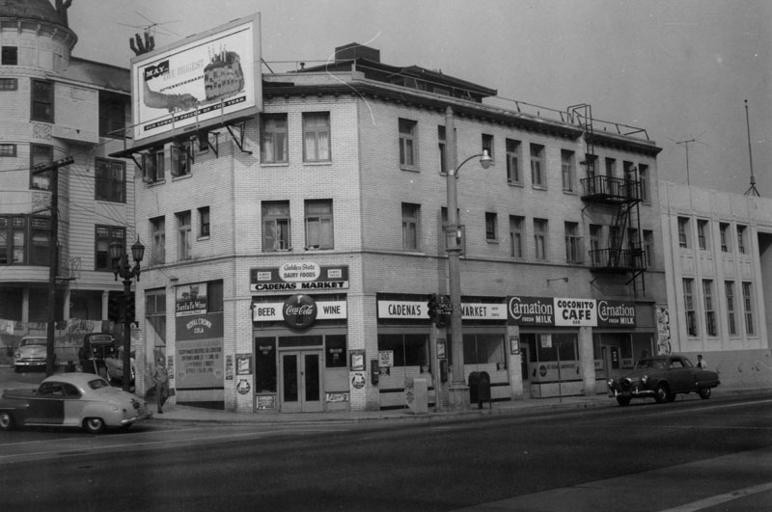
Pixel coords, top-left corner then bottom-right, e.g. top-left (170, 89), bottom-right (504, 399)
top-left (427, 294), bottom-right (453, 329)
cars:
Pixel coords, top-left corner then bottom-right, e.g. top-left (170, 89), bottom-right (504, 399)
top-left (0, 370), bottom-right (153, 433)
top-left (13, 335), bottom-right (48, 372)
top-left (78, 333), bottom-right (136, 384)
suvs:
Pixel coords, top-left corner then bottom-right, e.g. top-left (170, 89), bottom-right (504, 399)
top-left (608, 355), bottom-right (720, 406)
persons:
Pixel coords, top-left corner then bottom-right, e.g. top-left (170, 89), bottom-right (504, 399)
top-left (153, 357), bottom-right (168, 414)
top-left (695, 355), bottom-right (708, 368)
top-left (144, 82), bottom-right (196, 111)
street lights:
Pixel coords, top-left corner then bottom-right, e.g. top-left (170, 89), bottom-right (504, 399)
top-left (446, 150), bottom-right (493, 411)
top-left (108, 233), bottom-right (144, 391)
